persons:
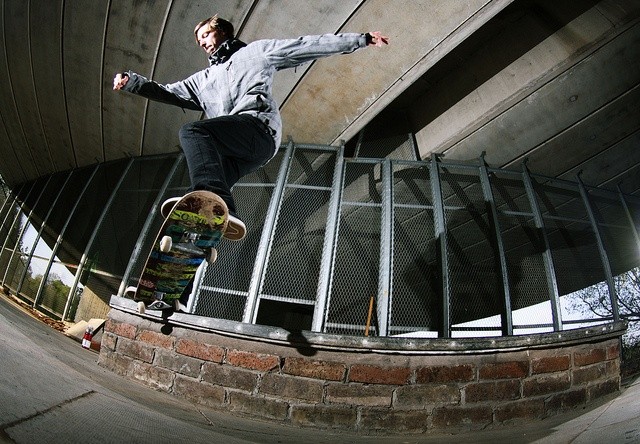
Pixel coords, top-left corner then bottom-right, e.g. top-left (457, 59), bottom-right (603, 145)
top-left (112, 14), bottom-right (388, 314)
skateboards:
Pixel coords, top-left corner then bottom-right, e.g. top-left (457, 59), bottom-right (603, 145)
top-left (133, 191), bottom-right (229, 313)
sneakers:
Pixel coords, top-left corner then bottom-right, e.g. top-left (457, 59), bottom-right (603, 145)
top-left (124, 285), bottom-right (138, 302)
top-left (160, 196), bottom-right (247, 242)
